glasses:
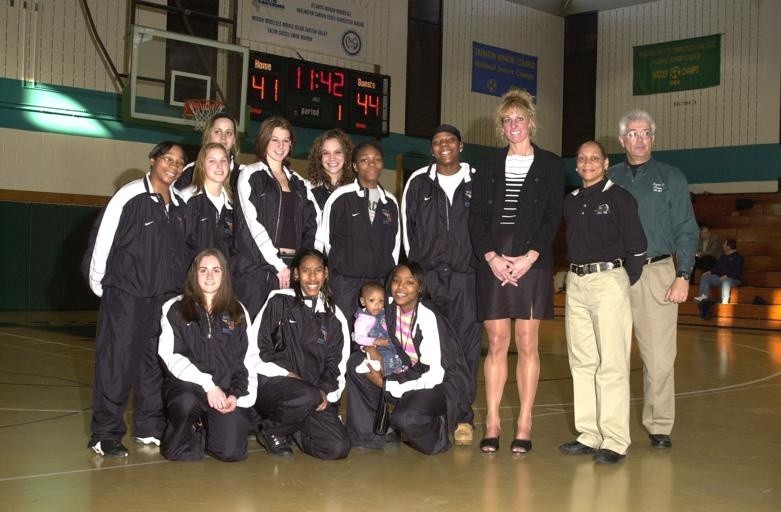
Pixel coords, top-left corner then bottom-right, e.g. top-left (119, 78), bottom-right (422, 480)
top-left (627, 129), bottom-right (653, 140)
top-left (162, 155), bottom-right (185, 165)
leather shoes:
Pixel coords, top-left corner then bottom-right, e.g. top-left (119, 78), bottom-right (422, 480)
top-left (90, 441), bottom-right (129, 458)
top-left (559, 441), bottom-right (591, 456)
top-left (594, 449), bottom-right (626, 462)
top-left (651, 434), bottom-right (671, 448)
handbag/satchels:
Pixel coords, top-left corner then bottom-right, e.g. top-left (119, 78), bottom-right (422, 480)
top-left (375, 377), bottom-right (389, 435)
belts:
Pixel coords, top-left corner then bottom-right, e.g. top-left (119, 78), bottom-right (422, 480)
top-left (569, 260), bottom-right (622, 275)
top-left (643, 255), bottom-right (671, 263)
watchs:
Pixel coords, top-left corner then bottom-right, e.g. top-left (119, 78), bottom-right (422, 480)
top-left (674, 271), bottom-right (693, 281)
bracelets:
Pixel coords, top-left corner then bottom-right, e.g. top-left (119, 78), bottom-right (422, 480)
top-left (523, 253), bottom-right (536, 267)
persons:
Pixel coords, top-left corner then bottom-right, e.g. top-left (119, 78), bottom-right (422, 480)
top-left (484, 253), bottom-right (498, 265)
top-left (692, 238), bottom-right (743, 304)
top-left (468, 87), bottom-right (565, 455)
top-left (689, 225), bottom-right (721, 285)
top-left (346, 261), bottom-right (475, 455)
top-left (157, 247), bottom-right (253, 465)
top-left (80, 140), bottom-right (187, 458)
top-left (400, 124), bottom-right (482, 407)
top-left (606, 109), bottom-right (700, 450)
top-left (556, 139), bottom-right (649, 465)
top-left (304, 128), bottom-right (356, 212)
top-left (177, 112), bottom-right (248, 191)
top-left (354, 281), bottom-right (409, 377)
top-left (250, 250), bottom-right (352, 461)
top-left (234, 117), bottom-right (320, 327)
top-left (177, 141), bottom-right (234, 254)
top-left (318, 141), bottom-right (402, 322)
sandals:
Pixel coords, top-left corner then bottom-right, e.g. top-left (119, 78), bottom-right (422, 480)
top-left (479, 437), bottom-right (499, 453)
top-left (511, 438), bottom-right (531, 454)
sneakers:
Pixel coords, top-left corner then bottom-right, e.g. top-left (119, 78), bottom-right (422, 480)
top-left (256, 430), bottom-right (293, 458)
top-left (454, 422), bottom-right (474, 444)
top-left (135, 437), bottom-right (161, 447)
top-left (693, 295), bottom-right (708, 301)
